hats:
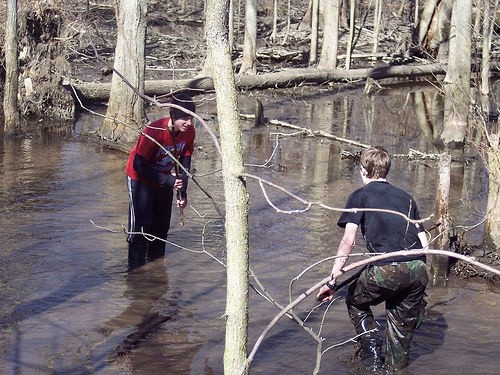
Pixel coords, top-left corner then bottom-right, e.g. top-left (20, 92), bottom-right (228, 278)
top-left (170, 93), bottom-right (195, 118)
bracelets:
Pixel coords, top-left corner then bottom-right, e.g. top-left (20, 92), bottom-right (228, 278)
top-left (326, 281), bottom-right (336, 290)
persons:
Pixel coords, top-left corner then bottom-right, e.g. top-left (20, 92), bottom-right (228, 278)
top-left (316, 146), bottom-right (430, 369)
top-left (125, 94), bottom-right (196, 266)
